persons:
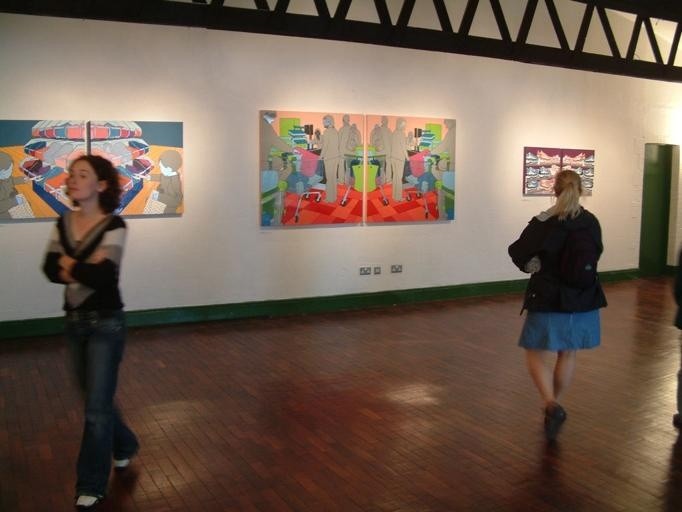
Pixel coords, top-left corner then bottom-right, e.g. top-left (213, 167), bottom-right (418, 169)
top-left (507, 169), bottom-right (610, 442)
top-left (672, 247), bottom-right (682, 430)
top-left (41, 155), bottom-right (140, 512)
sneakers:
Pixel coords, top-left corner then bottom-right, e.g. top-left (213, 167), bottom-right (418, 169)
top-left (74, 487), bottom-right (106, 512)
top-left (544, 401), bottom-right (567, 444)
top-left (112, 438), bottom-right (142, 476)
